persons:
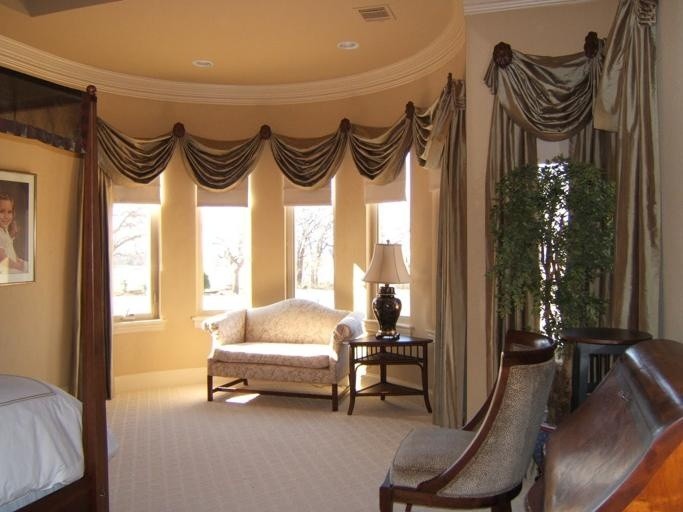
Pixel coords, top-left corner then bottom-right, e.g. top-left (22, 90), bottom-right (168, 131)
top-left (0, 192), bottom-right (25, 272)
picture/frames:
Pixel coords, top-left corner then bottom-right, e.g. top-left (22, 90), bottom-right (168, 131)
top-left (1, 168), bottom-right (37, 286)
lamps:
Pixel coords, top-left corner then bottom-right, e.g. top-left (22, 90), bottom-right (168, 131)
top-left (362, 239), bottom-right (411, 339)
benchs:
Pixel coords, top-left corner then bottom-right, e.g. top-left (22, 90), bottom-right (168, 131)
top-left (202, 299), bottom-right (363, 412)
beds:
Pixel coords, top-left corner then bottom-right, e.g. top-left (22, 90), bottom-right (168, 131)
top-left (1, 66), bottom-right (109, 512)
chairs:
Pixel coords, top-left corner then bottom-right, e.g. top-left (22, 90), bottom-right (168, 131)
top-left (379, 330), bottom-right (554, 511)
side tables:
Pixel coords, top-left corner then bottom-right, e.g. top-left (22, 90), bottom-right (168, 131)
top-left (348, 335), bottom-right (434, 416)
top-left (562, 328), bottom-right (652, 413)
